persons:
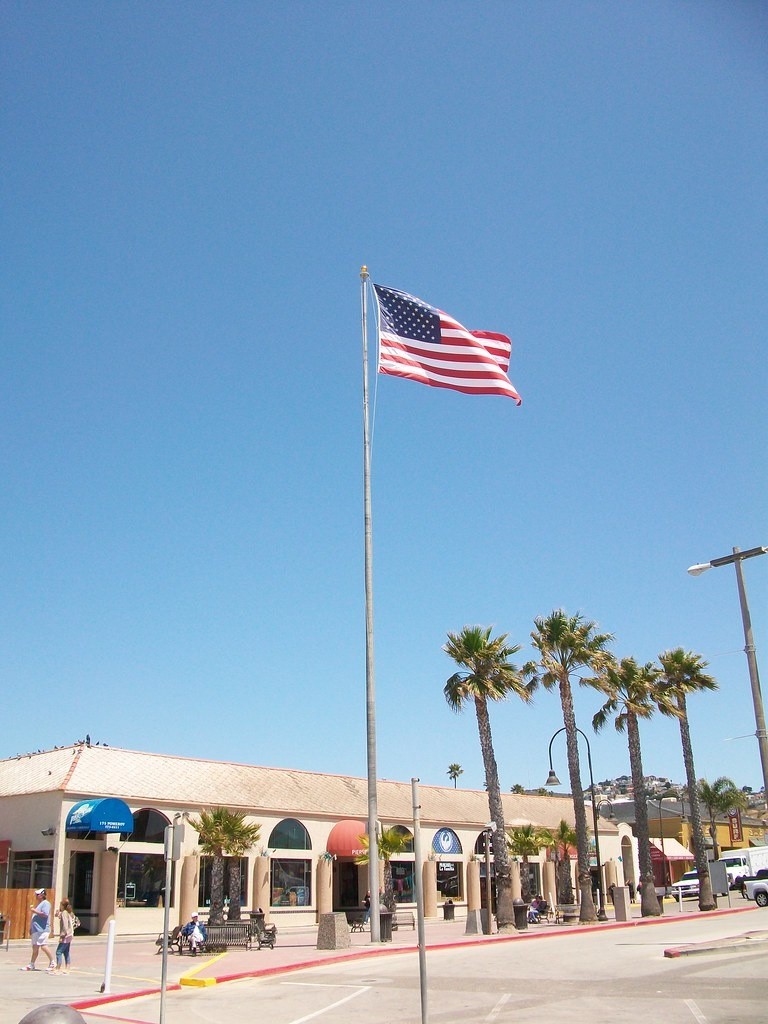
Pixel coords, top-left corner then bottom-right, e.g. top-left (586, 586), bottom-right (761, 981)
top-left (177, 912), bottom-right (207, 958)
top-left (527, 894), bottom-right (547, 924)
top-left (626, 880), bottom-right (635, 904)
top-left (19, 887), bottom-right (75, 976)
top-left (741, 875), bottom-right (750, 899)
top-left (607, 882), bottom-right (616, 905)
top-left (637, 882), bottom-right (642, 894)
top-left (362, 885), bottom-right (385, 925)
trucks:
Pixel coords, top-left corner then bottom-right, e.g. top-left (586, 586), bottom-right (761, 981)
top-left (717, 846), bottom-right (768, 889)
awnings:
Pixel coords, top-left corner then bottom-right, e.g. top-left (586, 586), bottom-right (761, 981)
top-left (649, 838), bottom-right (695, 863)
top-left (749, 838), bottom-right (767, 847)
top-left (326, 820), bottom-right (370, 856)
top-left (65, 797), bottom-right (134, 832)
top-left (704, 837), bottom-right (719, 846)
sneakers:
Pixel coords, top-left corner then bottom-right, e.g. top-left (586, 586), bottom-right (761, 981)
top-left (46, 962), bottom-right (56, 971)
top-left (20, 964), bottom-right (35, 971)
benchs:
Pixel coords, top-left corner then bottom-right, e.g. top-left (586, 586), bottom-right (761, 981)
top-left (154, 923), bottom-right (183, 955)
top-left (350, 911), bottom-right (367, 933)
top-left (390, 911), bottom-right (415, 930)
top-left (261, 916), bottom-right (277, 938)
top-left (179, 919), bottom-right (256, 954)
top-left (535, 904), bottom-right (551, 924)
top-left (254, 918), bottom-right (275, 950)
top-left (553, 904), bottom-right (599, 925)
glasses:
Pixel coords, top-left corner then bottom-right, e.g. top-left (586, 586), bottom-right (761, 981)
top-left (36, 894), bottom-right (40, 896)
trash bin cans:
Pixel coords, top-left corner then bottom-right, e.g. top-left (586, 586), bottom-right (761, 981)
top-left (380, 912), bottom-right (394, 942)
top-left (442, 899), bottom-right (456, 920)
top-left (713, 894), bottom-right (717, 908)
top-left (657, 896), bottom-right (664, 913)
top-left (513, 904), bottom-right (527, 930)
top-left (249, 912), bottom-right (265, 937)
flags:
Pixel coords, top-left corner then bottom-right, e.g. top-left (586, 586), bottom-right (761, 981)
top-left (372, 283), bottom-right (522, 406)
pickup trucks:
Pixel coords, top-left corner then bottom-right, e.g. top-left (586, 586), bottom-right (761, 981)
top-left (742, 876), bottom-right (768, 908)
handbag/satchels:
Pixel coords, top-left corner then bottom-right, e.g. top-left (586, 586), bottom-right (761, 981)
top-left (72, 915), bottom-right (80, 929)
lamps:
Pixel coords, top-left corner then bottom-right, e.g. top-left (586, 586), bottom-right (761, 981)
top-left (41, 826), bottom-right (55, 837)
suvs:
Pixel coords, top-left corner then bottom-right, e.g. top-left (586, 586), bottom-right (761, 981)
top-left (671, 871), bottom-right (701, 902)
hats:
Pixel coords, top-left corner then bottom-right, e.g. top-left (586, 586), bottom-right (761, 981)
top-left (191, 912), bottom-right (198, 918)
top-left (35, 889), bottom-right (46, 895)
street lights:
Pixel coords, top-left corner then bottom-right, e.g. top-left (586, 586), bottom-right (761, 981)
top-left (544, 726), bottom-right (619, 922)
top-left (659, 792), bottom-right (689, 898)
top-left (688, 546), bottom-right (768, 799)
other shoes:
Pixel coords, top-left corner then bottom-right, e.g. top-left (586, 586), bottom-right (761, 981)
top-left (192, 949), bottom-right (196, 957)
top-left (48, 966), bottom-right (62, 973)
top-left (63, 969), bottom-right (71, 974)
top-left (199, 943), bottom-right (204, 950)
